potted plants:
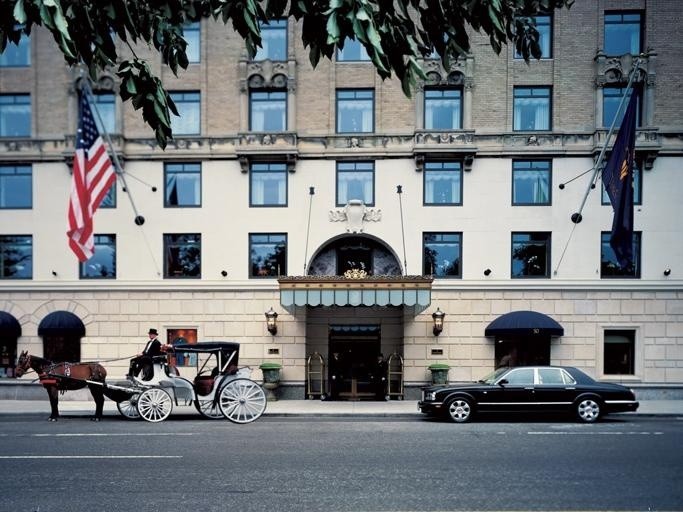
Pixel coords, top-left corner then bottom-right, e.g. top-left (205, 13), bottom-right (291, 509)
top-left (259, 363), bottom-right (283, 383)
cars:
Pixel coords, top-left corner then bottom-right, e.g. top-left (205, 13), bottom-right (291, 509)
top-left (417, 365), bottom-right (639, 422)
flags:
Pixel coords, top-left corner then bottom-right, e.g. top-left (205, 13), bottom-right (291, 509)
top-left (602, 73), bottom-right (640, 274)
top-left (67, 73), bottom-right (117, 264)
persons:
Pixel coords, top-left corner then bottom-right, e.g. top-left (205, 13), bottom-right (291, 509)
top-left (329, 349), bottom-right (346, 400)
top-left (372, 352), bottom-right (389, 401)
top-left (126, 328), bottom-right (162, 381)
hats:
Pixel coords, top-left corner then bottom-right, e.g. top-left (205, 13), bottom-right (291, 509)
top-left (148, 329), bottom-right (158, 335)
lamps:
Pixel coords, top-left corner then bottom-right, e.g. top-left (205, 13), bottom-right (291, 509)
top-left (265, 307), bottom-right (278, 336)
top-left (432, 307), bottom-right (445, 336)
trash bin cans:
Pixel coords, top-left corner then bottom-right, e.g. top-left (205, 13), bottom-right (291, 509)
top-left (258, 363), bottom-right (283, 401)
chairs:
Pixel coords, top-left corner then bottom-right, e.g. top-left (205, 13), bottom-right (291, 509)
top-left (194, 365), bottom-right (237, 395)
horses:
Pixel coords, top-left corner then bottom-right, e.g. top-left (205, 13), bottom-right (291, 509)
top-left (16, 351), bottom-right (107, 422)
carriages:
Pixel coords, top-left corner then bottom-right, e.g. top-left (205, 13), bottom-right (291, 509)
top-left (15, 341), bottom-right (264, 424)
